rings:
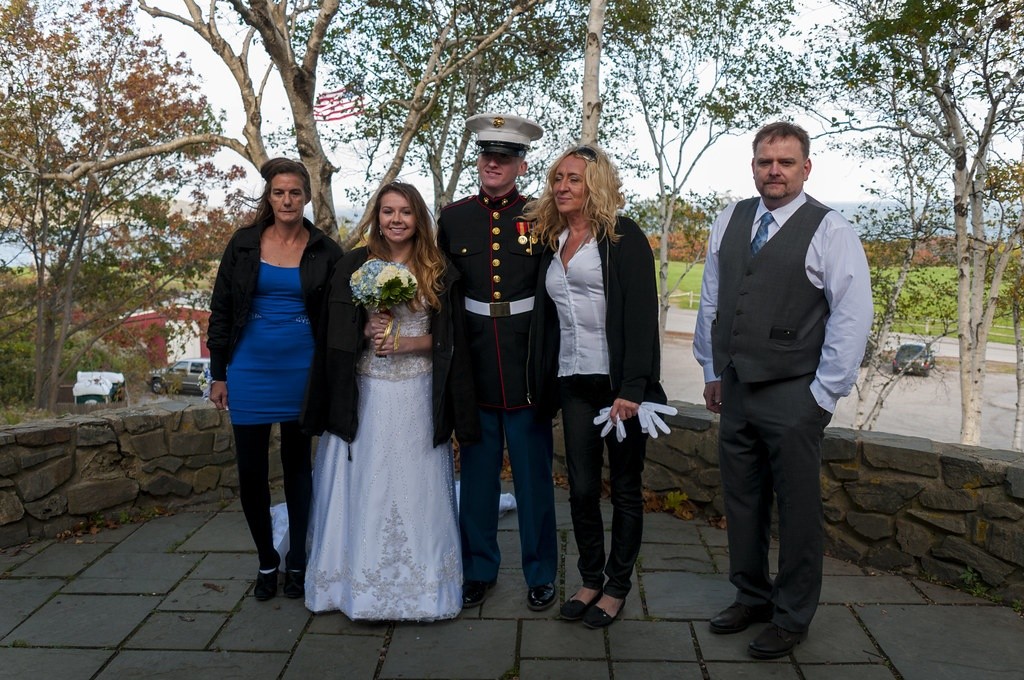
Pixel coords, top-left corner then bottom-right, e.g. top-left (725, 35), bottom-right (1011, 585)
top-left (380, 319), bottom-right (384, 324)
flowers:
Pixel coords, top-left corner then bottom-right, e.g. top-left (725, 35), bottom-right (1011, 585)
top-left (349, 259), bottom-right (419, 359)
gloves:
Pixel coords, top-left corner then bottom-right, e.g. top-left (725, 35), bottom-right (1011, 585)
top-left (593, 401), bottom-right (678, 442)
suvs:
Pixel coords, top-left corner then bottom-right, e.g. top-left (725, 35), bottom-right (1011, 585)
top-left (148, 359), bottom-right (211, 396)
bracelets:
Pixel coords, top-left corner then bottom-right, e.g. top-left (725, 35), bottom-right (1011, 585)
top-left (212, 381), bottom-right (216, 383)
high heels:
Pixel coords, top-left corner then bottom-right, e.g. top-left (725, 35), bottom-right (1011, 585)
top-left (254, 566), bottom-right (278, 600)
top-left (283, 552), bottom-right (308, 599)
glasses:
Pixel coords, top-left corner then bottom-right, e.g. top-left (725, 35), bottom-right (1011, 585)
top-left (567, 147), bottom-right (597, 164)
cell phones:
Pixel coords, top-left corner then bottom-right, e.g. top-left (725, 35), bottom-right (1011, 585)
top-left (769, 326), bottom-right (798, 340)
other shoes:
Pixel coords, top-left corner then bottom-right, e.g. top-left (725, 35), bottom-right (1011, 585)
top-left (582, 596), bottom-right (626, 629)
top-left (559, 587), bottom-right (603, 620)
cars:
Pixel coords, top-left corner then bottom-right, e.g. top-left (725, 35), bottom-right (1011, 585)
top-left (893, 345), bottom-right (934, 377)
top-left (859, 342), bottom-right (876, 366)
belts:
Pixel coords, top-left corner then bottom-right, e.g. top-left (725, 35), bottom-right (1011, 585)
top-left (465, 296), bottom-right (535, 317)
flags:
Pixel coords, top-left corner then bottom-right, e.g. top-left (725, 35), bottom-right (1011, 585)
top-left (314, 76), bottom-right (367, 121)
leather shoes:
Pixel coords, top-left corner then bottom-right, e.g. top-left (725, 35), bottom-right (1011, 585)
top-left (462, 579), bottom-right (497, 608)
top-left (526, 581), bottom-right (557, 611)
top-left (748, 623), bottom-right (809, 659)
top-left (709, 600), bottom-right (774, 634)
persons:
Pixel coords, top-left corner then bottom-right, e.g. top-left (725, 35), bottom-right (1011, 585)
top-left (304, 183), bottom-right (464, 622)
top-left (692, 122), bottom-right (875, 659)
top-left (526, 144), bottom-right (668, 630)
top-left (206, 158), bottom-right (344, 601)
top-left (437, 113), bottom-right (559, 612)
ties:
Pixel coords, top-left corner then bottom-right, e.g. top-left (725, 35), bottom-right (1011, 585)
top-left (751, 212), bottom-right (775, 246)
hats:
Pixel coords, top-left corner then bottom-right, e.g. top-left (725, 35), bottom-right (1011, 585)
top-left (465, 113), bottom-right (544, 157)
